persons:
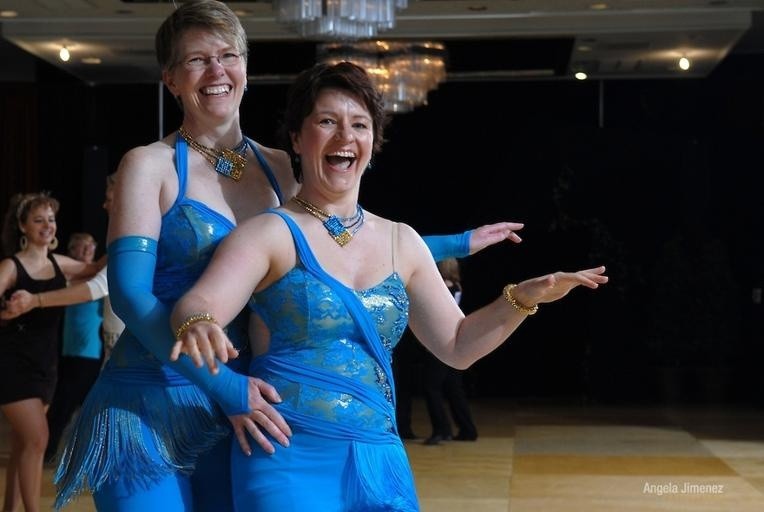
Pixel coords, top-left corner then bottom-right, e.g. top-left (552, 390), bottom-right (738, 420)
top-left (166, 60), bottom-right (609, 512)
top-left (393, 318), bottom-right (425, 440)
top-left (416, 252), bottom-right (481, 444)
top-left (0, 174), bottom-right (128, 512)
top-left (48, 0), bottom-right (528, 510)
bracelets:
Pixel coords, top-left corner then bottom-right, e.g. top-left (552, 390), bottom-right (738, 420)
top-left (500, 282), bottom-right (540, 316)
top-left (171, 312), bottom-right (220, 339)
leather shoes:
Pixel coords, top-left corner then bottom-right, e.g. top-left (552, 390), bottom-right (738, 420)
top-left (399, 426), bottom-right (422, 440)
top-left (424, 424), bottom-right (480, 447)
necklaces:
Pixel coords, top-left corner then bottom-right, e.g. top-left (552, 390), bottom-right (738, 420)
top-left (290, 196), bottom-right (367, 248)
top-left (177, 128), bottom-right (249, 183)
